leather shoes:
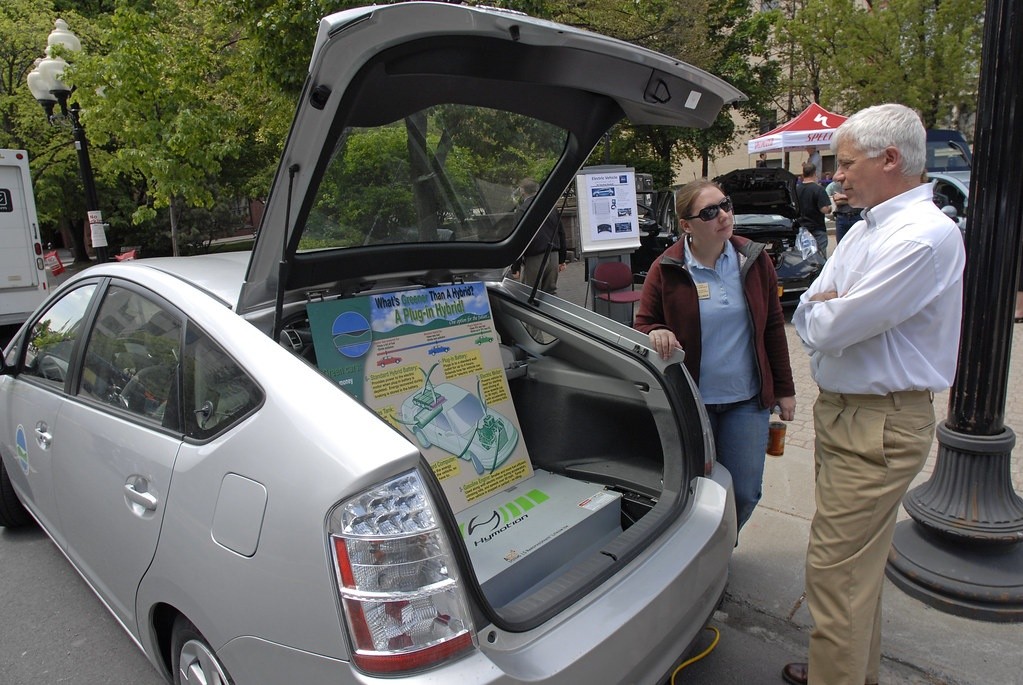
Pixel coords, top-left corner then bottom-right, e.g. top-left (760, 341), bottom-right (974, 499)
top-left (782, 661), bottom-right (808, 685)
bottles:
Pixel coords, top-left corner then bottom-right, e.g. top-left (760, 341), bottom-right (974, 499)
top-left (766, 405), bottom-right (788, 457)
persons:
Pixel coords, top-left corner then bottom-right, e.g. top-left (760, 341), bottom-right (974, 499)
top-left (511, 179), bottom-right (566, 296)
top-left (780, 102), bottom-right (966, 685)
top-left (756, 152), bottom-right (768, 168)
top-left (826, 177), bottom-right (864, 246)
top-left (802, 145), bottom-right (822, 185)
top-left (796, 163), bottom-right (832, 289)
top-left (634, 177), bottom-right (797, 547)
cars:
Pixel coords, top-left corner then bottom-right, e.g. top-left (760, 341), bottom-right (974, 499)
top-left (707, 166), bottom-right (829, 310)
top-left (632, 183), bottom-right (690, 266)
top-left (0, 0), bottom-right (738, 684)
top-left (923, 170), bottom-right (971, 242)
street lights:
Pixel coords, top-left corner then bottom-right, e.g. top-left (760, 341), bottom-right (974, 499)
top-left (26, 15), bottom-right (111, 266)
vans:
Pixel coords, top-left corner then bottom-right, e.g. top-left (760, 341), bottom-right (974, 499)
top-left (924, 127), bottom-right (974, 172)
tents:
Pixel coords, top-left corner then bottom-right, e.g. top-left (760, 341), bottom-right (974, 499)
top-left (747, 102), bottom-right (851, 168)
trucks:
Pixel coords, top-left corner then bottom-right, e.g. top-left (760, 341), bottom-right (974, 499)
top-left (0, 149), bottom-right (51, 324)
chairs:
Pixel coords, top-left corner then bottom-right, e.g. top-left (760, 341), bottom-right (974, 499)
top-left (590, 254), bottom-right (642, 328)
top-left (117, 311), bottom-right (179, 413)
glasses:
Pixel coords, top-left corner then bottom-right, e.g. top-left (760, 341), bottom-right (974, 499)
top-left (683, 196), bottom-right (732, 222)
